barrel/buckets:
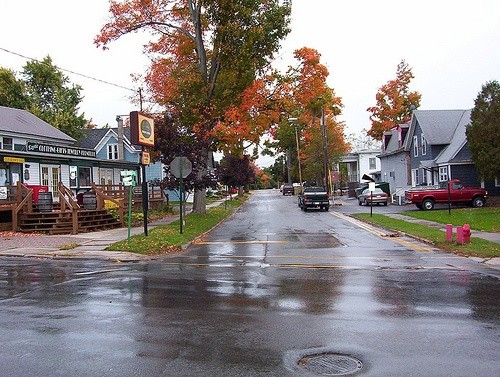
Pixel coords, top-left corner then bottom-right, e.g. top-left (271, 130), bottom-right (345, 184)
top-left (83, 192), bottom-right (97, 210)
top-left (38, 192), bottom-right (53, 213)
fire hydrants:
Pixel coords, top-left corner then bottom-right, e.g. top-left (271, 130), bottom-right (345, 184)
top-left (463, 224), bottom-right (471, 242)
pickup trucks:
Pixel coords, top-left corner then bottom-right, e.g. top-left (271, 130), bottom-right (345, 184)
top-left (283, 183), bottom-right (294, 195)
top-left (405, 179), bottom-right (488, 211)
top-left (298, 187), bottom-right (329, 211)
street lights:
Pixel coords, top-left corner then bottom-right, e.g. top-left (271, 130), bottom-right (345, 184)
top-left (288, 118), bottom-right (303, 193)
top-left (317, 95), bottom-right (328, 191)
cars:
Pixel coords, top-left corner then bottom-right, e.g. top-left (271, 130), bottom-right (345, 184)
top-left (358, 188), bottom-right (388, 206)
